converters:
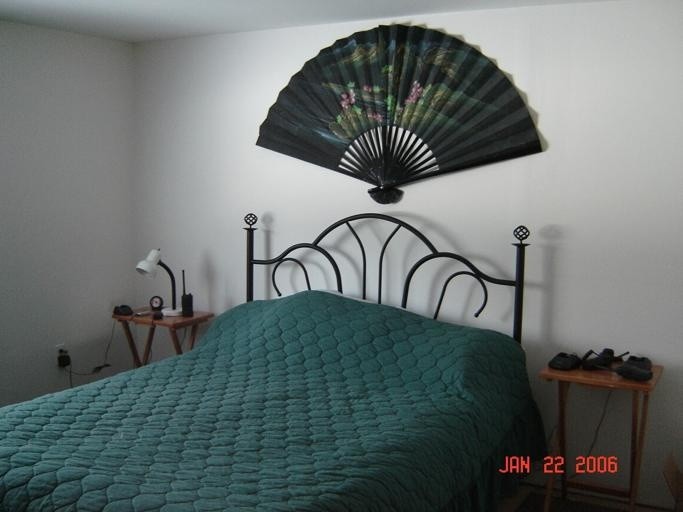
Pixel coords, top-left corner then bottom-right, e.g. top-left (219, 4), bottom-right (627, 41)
top-left (58, 355), bottom-right (71, 367)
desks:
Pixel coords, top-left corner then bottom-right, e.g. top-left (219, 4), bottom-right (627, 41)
top-left (537, 358), bottom-right (664, 511)
top-left (111, 305), bottom-right (214, 368)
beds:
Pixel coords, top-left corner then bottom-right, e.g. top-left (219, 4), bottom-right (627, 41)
top-left (0, 208), bottom-right (541, 509)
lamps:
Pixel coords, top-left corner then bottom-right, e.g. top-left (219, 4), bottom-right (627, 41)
top-left (135, 248), bottom-right (185, 317)
